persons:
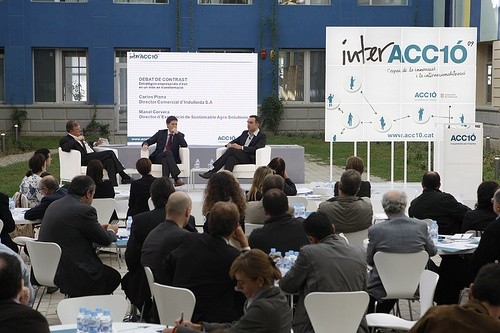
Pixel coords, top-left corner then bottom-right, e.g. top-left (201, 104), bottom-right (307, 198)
top-left (0, 149), bottom-right (500, 333)
top-left (60, 120), bottom-right (136, 186)
top-left (142, 116), bottom-right (188, 186)
top-left (198, 115), bottom-right (266, 179)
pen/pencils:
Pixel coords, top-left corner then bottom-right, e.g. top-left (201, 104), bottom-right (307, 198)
top-left (181, 312), bottom-right (184, 322)
top-left (461, 235), bottom-right (463, 238)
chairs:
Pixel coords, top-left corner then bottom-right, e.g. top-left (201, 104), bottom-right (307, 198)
top-left (286, 195), bottom-right (309, 210)
top-left (291, 290), bottom-right (371, 333)
top-left (55, 141), bottom-right (120, 187)
top-left (24, 238), bottom-right (61, 309)
top-left (55, 294), bottom-right (128, 325)
top-left (151, 280), bottom-right (197, 326)
top-left (139, 143), bottom-right (190, 193)
top-left (370, 249), bottom-right (429, 312)
top-left (214, 144), bottom-right (272, 179)
top-left (11, 236), bottom-right (33, 252)
top-left (244, 221), bottom-right (264, 233)
top-left (144, 265), bottom-right (155, 287)
top-left (88, 198), bottom-right (124, 269)
top-left (340, 228), bottom-right (370, 253)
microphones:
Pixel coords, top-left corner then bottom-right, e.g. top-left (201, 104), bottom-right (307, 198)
top-left (449, 106), bottom-right (451, 123)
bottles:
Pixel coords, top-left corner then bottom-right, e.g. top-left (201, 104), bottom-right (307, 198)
top-left (268, 248), bottom-right (299, 287)
top-left (8, 198), bottom-right (15, 219)
top-left (426, 221), bottom-right (439, 248)
top-left (293, 203), bottom-right (306, 219)
top-left (76, 307), bottom-right (113, 333)
top-left (210, 159), bottom-right (215, 168)
top-left (126, 215), bottom-right (133, 240)
top-left (196, 159), bottom-right (200, 165)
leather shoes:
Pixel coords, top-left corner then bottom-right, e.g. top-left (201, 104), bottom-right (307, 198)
top-left (199, 170), bottom-right (214, 178)
top-left (174, 180), bottom-right (184, 187)
top-left (122, 176), bottom-right (133, 183)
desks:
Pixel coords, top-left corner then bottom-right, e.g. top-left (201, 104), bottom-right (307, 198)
top-left (432, 233), bottom-right (481, 256)
top-left (92, 223), bottom-right (130, 250)
top-left (8, 207), bottom-right (42, 224)
top-left (48, 322), bottom-right (175, 333)
top-left (122, 167), bottom-right (143, 179)
top-left (190, 167), bottom-right (212, 191)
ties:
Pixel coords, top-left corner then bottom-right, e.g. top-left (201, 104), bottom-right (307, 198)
top-left (80, 140), bottom-right (86, 149)
top-left (166, 134), bottom-right (173, 152)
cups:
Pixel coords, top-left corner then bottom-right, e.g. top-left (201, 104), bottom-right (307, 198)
top-left (208, 164), bottom-right (212, 170)
top-left (195, 164), bottom-right (199, 170)
